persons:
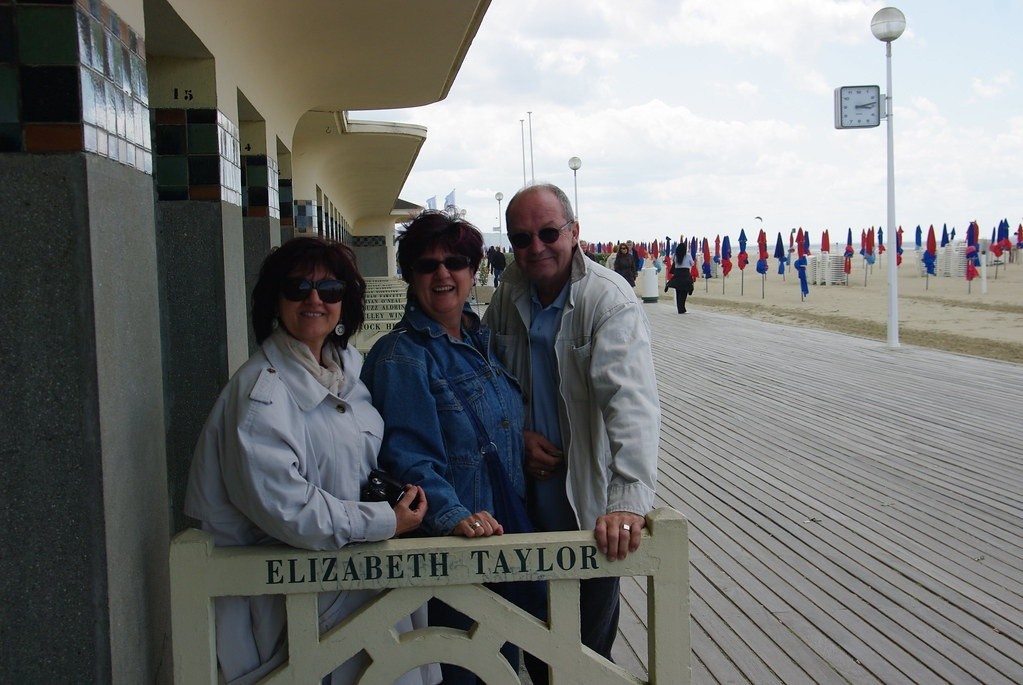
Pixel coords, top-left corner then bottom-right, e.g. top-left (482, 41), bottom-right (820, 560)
top-left (579, 241), bottom-right (595, 262)
top-left (359, 211), bottom-right (528, 685)
top-left (185, 237), bottom-right (443, 685)
top-left (664, 243), bottom-right (695, 314)
top-left (626, 240), bottom-right (639, 280)
top-left (486, 246), bottom-right (495, 274)
top-left (615, 243), bottom-right (636, 288)
top-left (480, 183), bottom-right (660, 685)
top-left (492, 247), bottom-right (506, 288)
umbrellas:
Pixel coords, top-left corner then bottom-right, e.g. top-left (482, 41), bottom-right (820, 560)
top-left (579, 235), bottom-right (699, 301)
top-left (699, 237), bottom-right (711, 293)
top-left (738, 229), bottom-right (749, 296)
top-left (756, 229), bottom-right (768, 299)
top-left (895, 218), bottom-right (1023, 294)
top-left (774, 233), bottom-right (787, 280)
top-left (714, 234), bottom-right (721, 278)
top-left (820, 229), bottom-right (830, 253)
top-left (787, 232), bottom-right (795, 272)
top-left (860, 226), bottom-right (885, 287)
top-left (844, 228), bottom-right (854, 286)
top-left (795, 227), bottom-right (811, 302)
top-left (721, 236), bottom-right (732, 294)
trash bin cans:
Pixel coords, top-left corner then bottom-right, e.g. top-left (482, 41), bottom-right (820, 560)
top-left (640, 266), bottom-right (658, 302)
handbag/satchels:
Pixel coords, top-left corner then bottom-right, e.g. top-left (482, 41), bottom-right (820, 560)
top-left (490, 476), bottom-right (536, 601)
top-left (360, 468), bottom-right (438, 538)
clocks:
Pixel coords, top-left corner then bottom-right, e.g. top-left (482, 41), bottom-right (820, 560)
top-left (833, 85), bottom-right (880, 129)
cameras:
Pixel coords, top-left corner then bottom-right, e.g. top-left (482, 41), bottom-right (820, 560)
top-left (359, 465), bottom-right (418, 512)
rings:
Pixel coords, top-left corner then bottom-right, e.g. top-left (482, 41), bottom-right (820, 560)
top-left (471, 522), bottom-right (481, 530)
top-left (622, 524), bottom-right (630, 530)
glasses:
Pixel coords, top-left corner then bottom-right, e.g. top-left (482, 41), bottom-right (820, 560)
top-left (287, 277), bottom-right (347, 303)
top-left (410, 255), bottom-right (472, 274)
top-left (509, 220), bottom-right (574, 248)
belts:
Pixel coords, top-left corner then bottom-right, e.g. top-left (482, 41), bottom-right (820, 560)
top-left (531, 465), bottom-right (554, 481)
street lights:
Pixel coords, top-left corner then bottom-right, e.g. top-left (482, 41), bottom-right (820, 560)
top-left (569, 157), bottom-right (582, 222)
top-left (871, 7), bottom-right (907, 348)
top-left (495, 192), bottom-right (504, 253)
